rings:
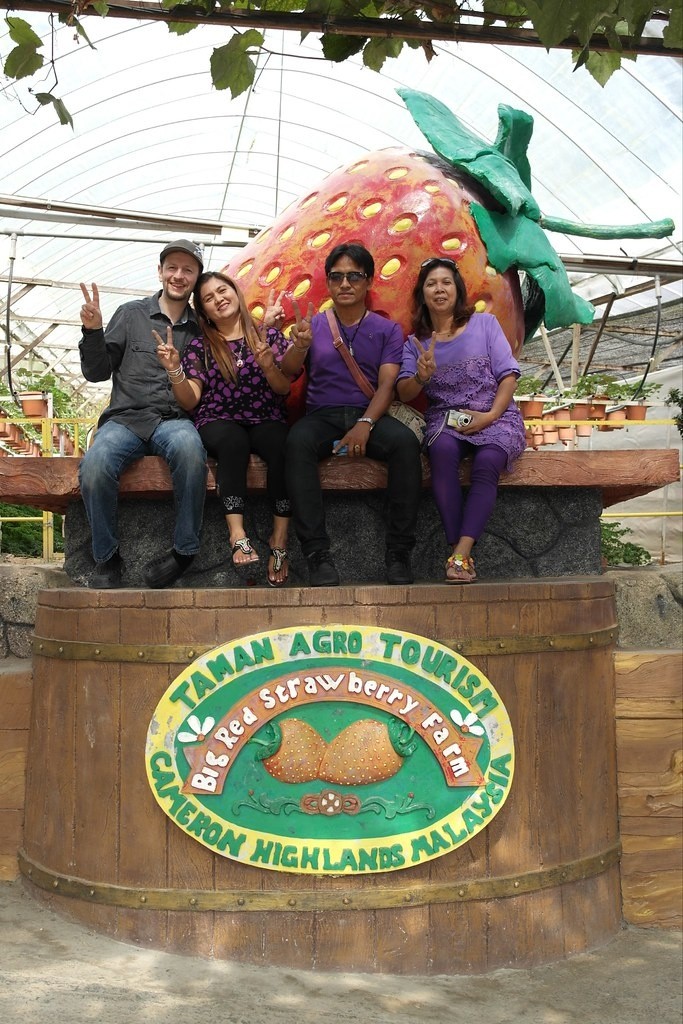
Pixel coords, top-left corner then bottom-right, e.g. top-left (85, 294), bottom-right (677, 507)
top-left (355, 445), bottom-right (360, 449)
top-left (306, 319), bottom-right (311, 323)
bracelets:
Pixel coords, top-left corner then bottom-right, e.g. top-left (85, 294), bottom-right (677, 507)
top-left (414, 371), bottom-right (431, 385)
top-left (165, 364), bottom-right (185, 385)
top-left (293, 344), bottom-right (310, 353)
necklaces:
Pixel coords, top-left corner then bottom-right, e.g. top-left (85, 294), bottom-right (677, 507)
top-left (231, 339), bottom-right (244, 368)
top-left (333, 307), bottom-right (368, 356)
top-left (436, 331), bottom-right (451, 336)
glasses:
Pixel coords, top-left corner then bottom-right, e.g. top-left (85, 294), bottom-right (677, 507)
top-left (326, 271), bottom-right (368, 283)
top-left (421, 257), bottom-right (457, 270)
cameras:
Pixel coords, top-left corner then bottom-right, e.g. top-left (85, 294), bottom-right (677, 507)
top-left (447, 409), bottom-right (472, 427)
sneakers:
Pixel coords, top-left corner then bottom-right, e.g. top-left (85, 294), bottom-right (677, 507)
top-left (307, 547), bottom-right (341, 585)
top-left (385, 547), bottom-right (413, 585)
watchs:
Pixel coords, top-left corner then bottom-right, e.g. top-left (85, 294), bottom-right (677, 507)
top-left (357, 418), bottom-right (375, 430)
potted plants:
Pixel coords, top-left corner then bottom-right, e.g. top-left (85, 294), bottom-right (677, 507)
top-left (0, 368), bottom-right (86, 458)
top-left (600, 520), bottom-right (651, 568)
top-left (515, 372), bottom-right (662, 446)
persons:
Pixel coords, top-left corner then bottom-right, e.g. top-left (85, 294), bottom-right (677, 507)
top-left (151, 271), bottom-right (313, 588)
top-left (394, 258), bottom-right (528, 584)
top-left (77, 242), bottom-right (285, 589)
top-left (280, 244), bottom-right (422, 585)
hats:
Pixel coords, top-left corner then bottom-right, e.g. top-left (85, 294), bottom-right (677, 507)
top-left (160, 239), bottom-right (203, 264)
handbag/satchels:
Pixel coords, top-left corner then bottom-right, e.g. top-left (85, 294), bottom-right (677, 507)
top-left (385, 401), bottom-right (427, 446)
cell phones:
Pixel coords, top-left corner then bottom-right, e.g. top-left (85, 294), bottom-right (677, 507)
top-left (334, 440), bottom-right (349, 453)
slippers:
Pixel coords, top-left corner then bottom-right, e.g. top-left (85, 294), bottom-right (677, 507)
top-left (265, 536), bottom-right (291, 588)
top-left (143, 548), bottom-right (190, 588)
top-left (230, 536), bottom-right (260, 567)
top-left (445, 554), bottom-right (478, 583)
top-left (93, 552), bottom-right (121, 589)
